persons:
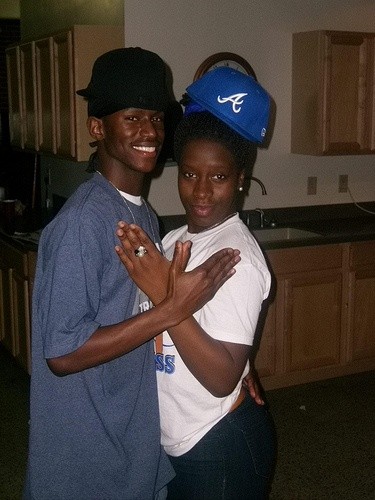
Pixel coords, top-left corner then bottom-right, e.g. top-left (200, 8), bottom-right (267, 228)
top-left (112, 89), bottom-right (281, 500)
top-left (21, 45), bottom-right (266, 500)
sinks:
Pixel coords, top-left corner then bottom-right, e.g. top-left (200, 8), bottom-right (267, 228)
top-left (248, 227), bottom-right (323, 243)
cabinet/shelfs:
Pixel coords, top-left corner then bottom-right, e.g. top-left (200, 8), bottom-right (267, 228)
top-left (291, 30), bottom-right (375, 156)
top-left (347, 242), bottom-right (375, 361)
top-left (250, 244), bottom-right (343, 377)
top-left (0, 240), bottom-right (31, 376)
top-left (6, 25), bottom-right (125, 162)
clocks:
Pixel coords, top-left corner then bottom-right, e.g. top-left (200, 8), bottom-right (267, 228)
top-left (193, 51), bottom-right (257, 83)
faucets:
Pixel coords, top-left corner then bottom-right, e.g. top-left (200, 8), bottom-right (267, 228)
top-left (245, 176), bottom-right (267, 196)
top-left (256, 209), bottom-right (265, 228)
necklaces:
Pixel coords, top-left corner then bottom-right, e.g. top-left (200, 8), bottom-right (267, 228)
top-left (96, 169), bottom-right (156, 245)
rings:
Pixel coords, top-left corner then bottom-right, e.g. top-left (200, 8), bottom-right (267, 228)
top-left (134, 246), bottom-right (148, 257)
